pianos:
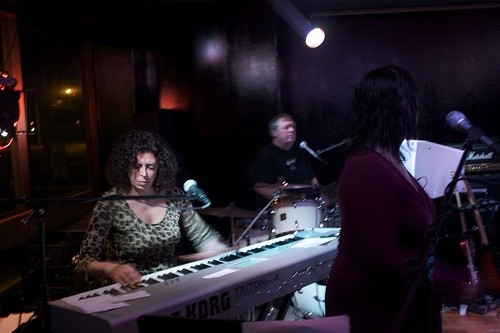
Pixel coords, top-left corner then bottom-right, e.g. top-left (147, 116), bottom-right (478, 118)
top-left (433, 137), bottom-right (500, 198)
top-left (47, 227), bottom-right (340, 333)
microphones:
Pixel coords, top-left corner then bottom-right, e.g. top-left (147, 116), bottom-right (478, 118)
top-left (445, 110), bottom-right (500, 154)
top-left (299, 141), bottom-right (321, 160)
top-left (183, 179), bottom-right (211, 205)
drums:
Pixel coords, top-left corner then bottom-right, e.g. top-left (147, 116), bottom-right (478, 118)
top-left (266, 197), bottom-right (327, 238)
top-left (230, 220), bottom-right (271, 247)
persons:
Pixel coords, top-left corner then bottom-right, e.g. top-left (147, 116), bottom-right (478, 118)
top-left (254, 113), bottom-right (319, 202)
top-left (72, 128), bottom-right (230, 286)
top-left (325, 64), bottom-right (442, 333)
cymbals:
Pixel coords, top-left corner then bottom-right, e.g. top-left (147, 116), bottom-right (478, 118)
top-left (199, 207), bottom-right (266, 218)
top-left (245, 182), bottom-right (319, 191)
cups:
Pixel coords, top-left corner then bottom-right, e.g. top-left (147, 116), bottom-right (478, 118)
top-left (459, 304), bottom-right (468, 316)
